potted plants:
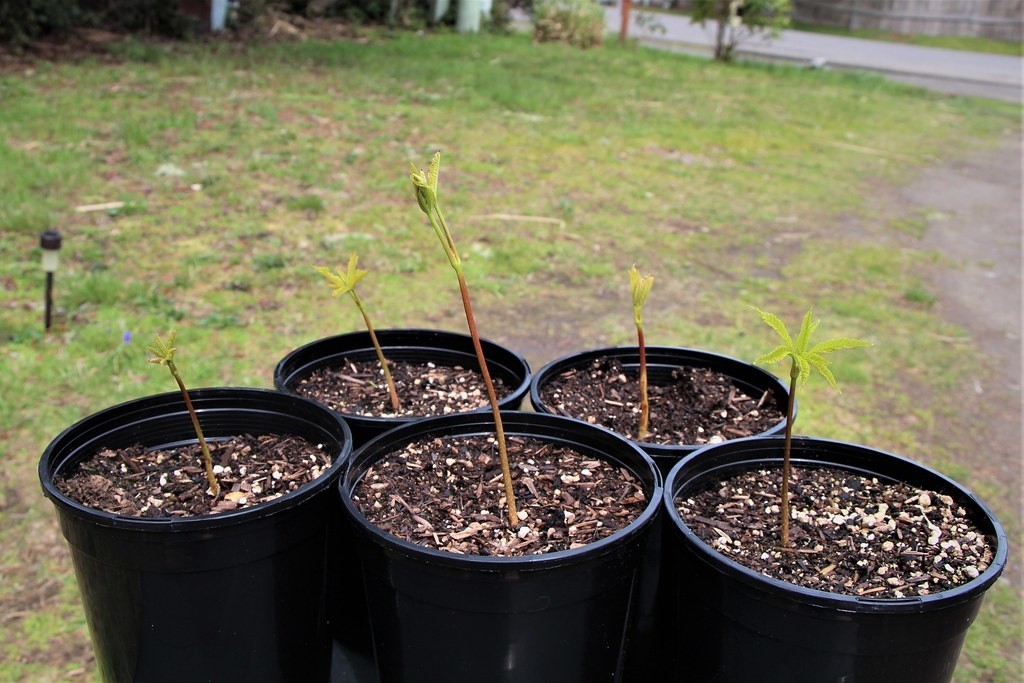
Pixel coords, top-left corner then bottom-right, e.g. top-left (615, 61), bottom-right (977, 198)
top-left (660, 302), bottom-right (1010, 683)
top-left (37, 328), bottom-right (353, 683)
top-left (530, 261), bottom-right (798, 670)
top-left (273, 253), bottom-right (531, 659)
top-left (337, 150), bottom-right (664, 683)
